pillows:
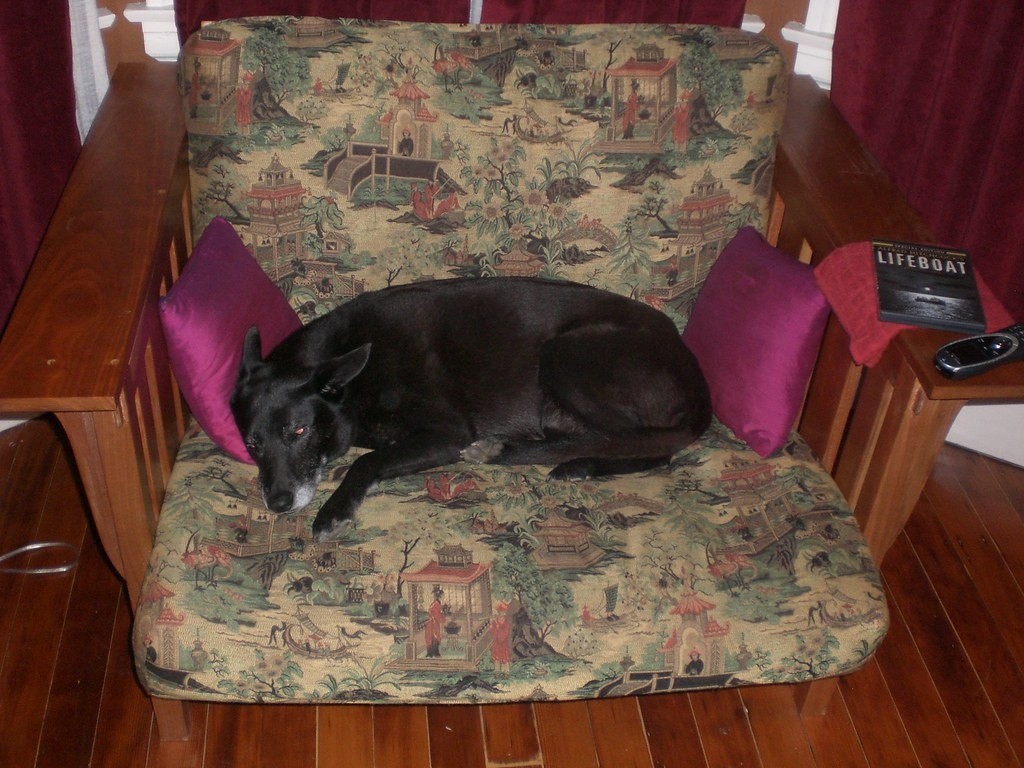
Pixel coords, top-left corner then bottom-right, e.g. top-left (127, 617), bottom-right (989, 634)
top-left (159, 216), bottom-right (303, 465)
top-left (681, 227), bottom-right (832, 457)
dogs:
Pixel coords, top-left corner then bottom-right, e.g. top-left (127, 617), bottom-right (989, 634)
top-left (229, 275), bottom-right (712, 541)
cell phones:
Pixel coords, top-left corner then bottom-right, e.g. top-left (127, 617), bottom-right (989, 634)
top-left (932, 322), bottom-right (1024, 381)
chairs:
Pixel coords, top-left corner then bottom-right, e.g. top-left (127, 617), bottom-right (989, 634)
top-left (0, 0), bottom-right (1024, 745)
